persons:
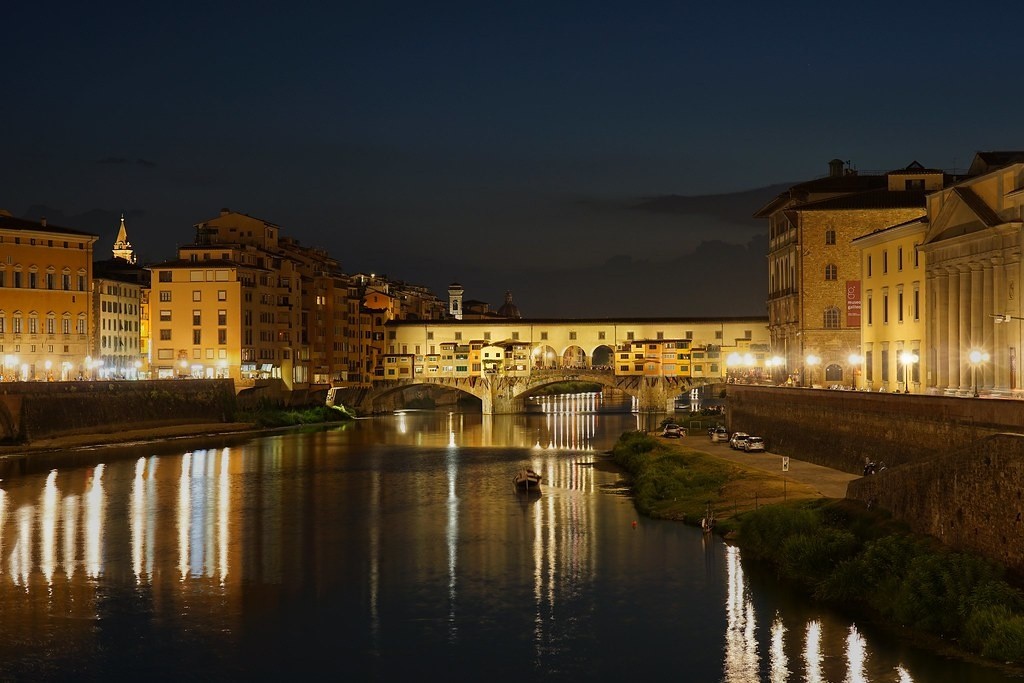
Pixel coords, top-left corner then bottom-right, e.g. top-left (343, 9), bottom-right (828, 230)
top-left (75, 376), bottom-right (96, 381)
top-left (864, 457), bottom-right (888, 476)
top-left (531, 365), bottom-right (614, 370)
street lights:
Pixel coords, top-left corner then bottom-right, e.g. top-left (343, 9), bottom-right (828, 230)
top-left (899, 350), bottom-right (919, 394)
top-left (805, 353), bottom-right (822, 389)
top-left (966, 345), bottom-right (992, 397)
top-left (845, 350), bottom-right (865, 391)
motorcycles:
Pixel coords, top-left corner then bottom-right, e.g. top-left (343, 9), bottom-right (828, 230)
top-left (863, 464), bottom-right (888, 475)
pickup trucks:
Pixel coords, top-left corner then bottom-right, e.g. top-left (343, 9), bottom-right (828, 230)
top-left (664, 423), bottom-right (680, 438)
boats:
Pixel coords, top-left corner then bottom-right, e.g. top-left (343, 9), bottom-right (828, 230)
top-left (512, 468), bottom-right (542, 494)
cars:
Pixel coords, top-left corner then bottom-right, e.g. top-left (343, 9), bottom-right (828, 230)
top-left (732, 435), bottom-right (750, 449)
top-left (729, 431), bottom-right (746, 447)
top-left (744, 436), bottom-right (766, 454)
top-left (680, 427), bottom-right (685, 438)
top-left (708, 426), bottom-right (728, 442)
